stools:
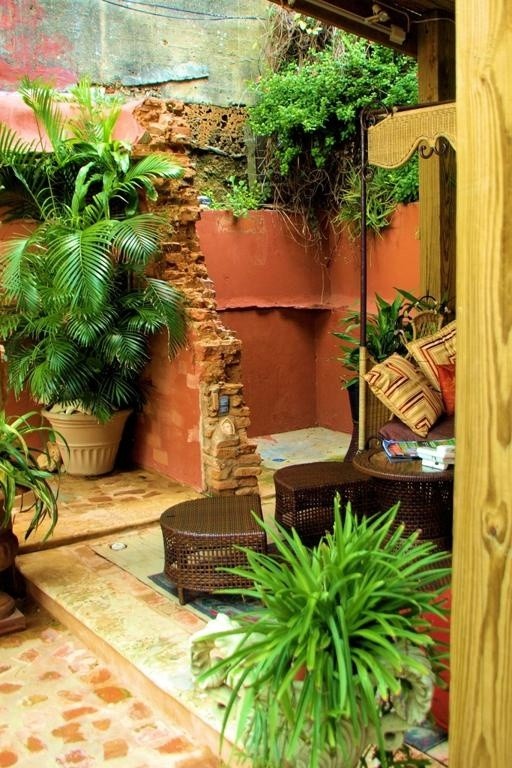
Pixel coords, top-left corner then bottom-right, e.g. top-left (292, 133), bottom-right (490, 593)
top-left (273, 463), bottom-right (371, 544)
top-left (159, 494), bottom-right (267, 607)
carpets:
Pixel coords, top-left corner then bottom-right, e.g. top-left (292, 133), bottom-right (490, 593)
top-left (84, 496), bottom-right (449, 767)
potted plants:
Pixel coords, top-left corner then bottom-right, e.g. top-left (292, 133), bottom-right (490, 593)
top-left (0, 409), bottom-right (70, 616)
top-left (190, 491), bottom-right (450, 768)
top-left (0, 70), bottom-right (186, 479)
top-left (324, 291), bottom-right (421, 465)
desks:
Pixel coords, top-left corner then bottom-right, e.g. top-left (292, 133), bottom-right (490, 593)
top-left (350, 447), bottom-right (454, 592)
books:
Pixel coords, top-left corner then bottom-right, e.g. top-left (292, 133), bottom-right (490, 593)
top-left (381, 437), bottom-right (455, 460)
top-left (385, 454), bottom-right (422, 463)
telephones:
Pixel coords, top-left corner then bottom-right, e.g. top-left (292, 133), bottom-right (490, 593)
top-left (417, 445), bottom-right (454, 470)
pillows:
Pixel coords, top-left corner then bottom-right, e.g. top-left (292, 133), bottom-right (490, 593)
top-left (360, 319), bottom-right (456, 441)
